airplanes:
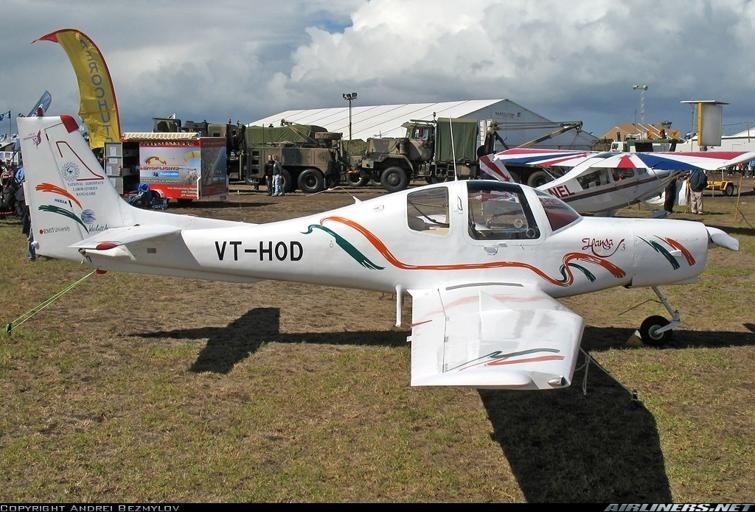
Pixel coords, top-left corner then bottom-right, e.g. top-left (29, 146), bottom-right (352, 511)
top-left (13, 111), bottom-right (742, 402)
top-left (482, 138), bottom-right (755, 217)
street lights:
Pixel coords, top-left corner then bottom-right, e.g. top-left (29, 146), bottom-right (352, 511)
top-left (342, 92), bottom-right (359, 139)
top-left (662, 120), bottom-right (671, 151)
top-left (632, 83), bottom-right (650, 124)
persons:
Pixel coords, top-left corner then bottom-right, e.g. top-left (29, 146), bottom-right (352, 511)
top-left (664, 178), bottom-right (677, 215)
top-left (271, 154), bottom-right (285, 196)
top-left (689, 169), bottom-right (708, 215)
top-left (264, 154), bottom-right (281, 196)
top-left (728, 159), bottom-right (755, 177)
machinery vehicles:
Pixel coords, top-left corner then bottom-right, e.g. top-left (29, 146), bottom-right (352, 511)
top-left (359, 110), bottom-right (585, 193)
top-left (145, 113), bottom-right (348, 193)
top-left (336, 138), bottom-right (373, 188)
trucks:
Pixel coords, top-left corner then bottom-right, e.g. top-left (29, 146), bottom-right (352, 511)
top-left (103, 129), bottom-right (231, 207)
